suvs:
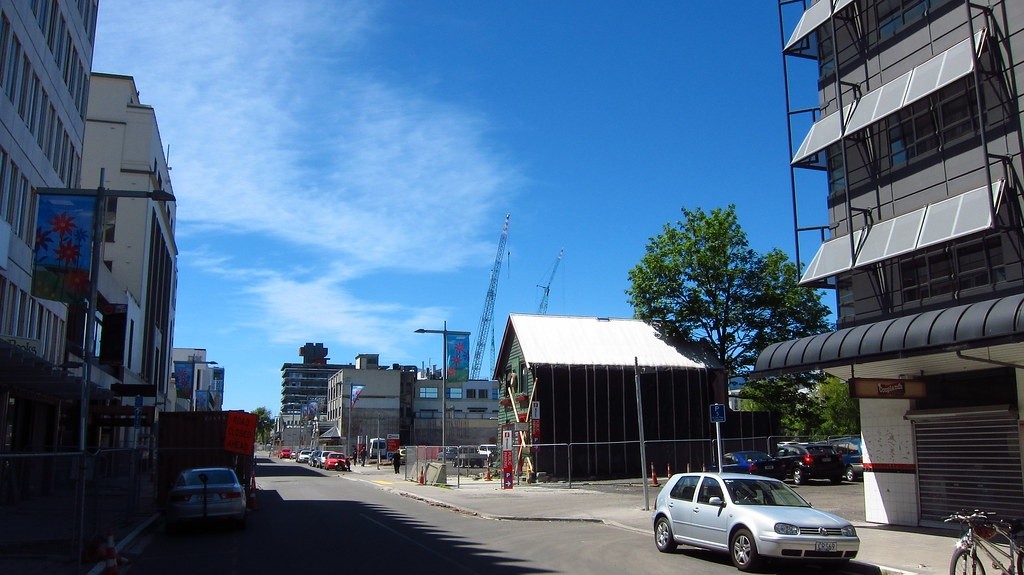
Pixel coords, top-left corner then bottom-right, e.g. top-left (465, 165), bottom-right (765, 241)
top-left (398, 448), bottom-right (415, 464)
top-left (438, 446), bottom-right (458, 461)
top-left (478, 444), bottom-right (497, 459)
top-left (774, 437), bottom-right (863, 485)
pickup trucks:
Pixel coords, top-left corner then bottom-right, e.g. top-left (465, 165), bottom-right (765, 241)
top-left (454, 446), bottom-right (487, 468)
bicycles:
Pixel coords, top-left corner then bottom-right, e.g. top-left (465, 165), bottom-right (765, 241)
top-left (944, 509), bottom-right (1024, 575)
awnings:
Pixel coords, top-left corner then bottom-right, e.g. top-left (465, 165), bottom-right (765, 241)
top-left (790, 28), bottom-right (996, 171)
top-left (755, 294), bottom-right (1024, 379)
top-left (782, 0), bottom-right (855, 60)
top-left (797, 179), bottom-right (1010, 289)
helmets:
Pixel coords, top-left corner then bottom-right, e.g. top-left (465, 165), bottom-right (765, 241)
top-left (974, 522), bottom-right (998, 539)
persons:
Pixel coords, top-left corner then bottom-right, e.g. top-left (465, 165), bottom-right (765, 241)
top-left (361, 446), bottom-right (367, 467)
top-left (353, 448), bottom-right (357, 466)
top-left (391, 450), bottom-right (401, 474)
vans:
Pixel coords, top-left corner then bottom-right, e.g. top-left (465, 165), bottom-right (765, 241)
top-left (369, 438), bottom-right (387, 458)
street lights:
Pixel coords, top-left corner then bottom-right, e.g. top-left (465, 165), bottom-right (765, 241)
top-left (284, 404), bottom-right (295, 447)
top-left (337, 382), bottom-right (353, 457)
top-left (79, 190), bottom-right (176, 452)
top-left (415, 328), bottom-right (446, 464)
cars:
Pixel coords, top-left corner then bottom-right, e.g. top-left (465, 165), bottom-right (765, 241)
top-left (652, 472), bottom-right (860, 572)
top-left (279, 448), bottom-right (346, 470)
top-left (708, 451), bottom-right (785, 480)
top-left (167, 466), bottom-right (246, 525)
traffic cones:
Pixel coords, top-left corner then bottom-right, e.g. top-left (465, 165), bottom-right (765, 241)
top-left (417, 465), bottom-right (425, 486)
top-left (92, 541), bottom-right (131, 565)
top-left (650, 462), bottom-right (661, 487)
top-left (102, 530), bottom-right (121, 575)
top-left (484, 462), bottom-right (492, 481)
